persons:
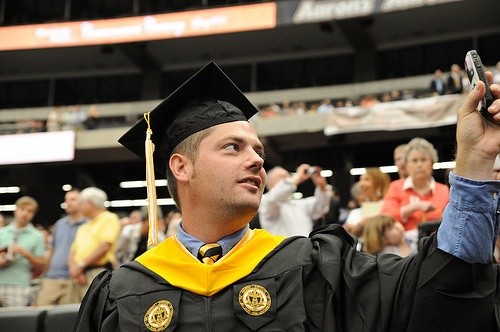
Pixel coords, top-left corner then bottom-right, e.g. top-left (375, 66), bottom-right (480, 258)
top-left (0, 195), bottom-right (46, 308)
top-left (66, 185), bottom-right (122, 305)
top-left (1, 136), bottom-right (500, 271)
top-left (45, 103), bottom-right (100, 133)
top-left (257, 63), bottom-right (474, 120)
top-left (71, 60), bottom-right (500, 332)
top-left (28, 186), bottom-right (84, 308)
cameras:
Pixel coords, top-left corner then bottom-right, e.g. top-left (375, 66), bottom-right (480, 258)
top-left (308, 167), bottom-right (314, 174)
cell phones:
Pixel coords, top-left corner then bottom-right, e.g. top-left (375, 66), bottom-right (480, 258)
top-left (465, 50), bottom-right (492, 111)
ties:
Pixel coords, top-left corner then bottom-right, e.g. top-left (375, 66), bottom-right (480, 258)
top-left (198, 242), bottom-right (223, 266)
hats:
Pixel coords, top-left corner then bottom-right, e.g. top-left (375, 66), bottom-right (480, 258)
top-left (118, 61), bottom-right (259, 247)
